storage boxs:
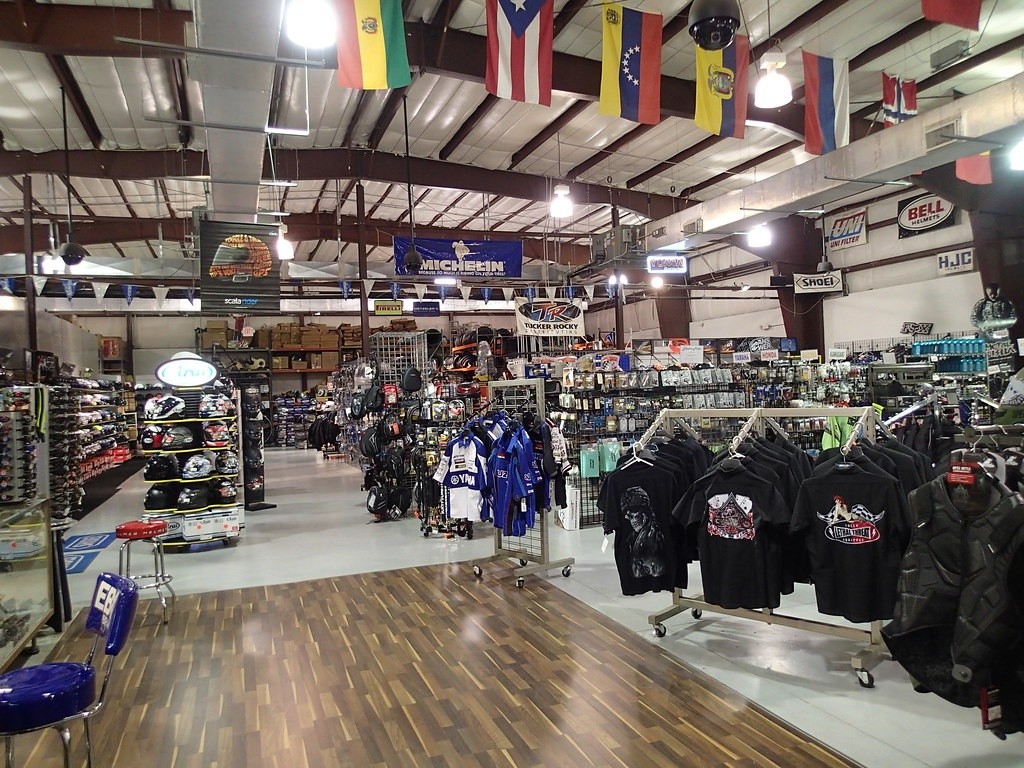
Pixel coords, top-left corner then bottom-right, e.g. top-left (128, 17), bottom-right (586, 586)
top-left (272, 318), bottom-right (338, 347)
top-left (289, 361), bottom-right (307, 370)
top-left (321, 351), bottom-right (339, 369)
top-left (202, 329), bottom-right (233, 349)
top-left (251, 329), bottom-right (271, 348)
top-left (206, 320), bottom-right (228, 332)
top-left (272, 356), bottom-right (289, 368)
top-left (306, 353), bottom-right (322, 369)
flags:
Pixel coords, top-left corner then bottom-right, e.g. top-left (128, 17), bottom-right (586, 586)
top-left (694, 35), bottom-right (749, 139)
top-left (484, 0), bottom-right (554, 108)
top-left (880, 72), bottom-right (917, 130)
top-left (954, 151), bottom-right (992, 185)
top-left (597, 1), bottom-right (662, 126)
top-left (801, 50), bottom-right (849, 155)
top-left (332, 0), bottom-right (413, 91)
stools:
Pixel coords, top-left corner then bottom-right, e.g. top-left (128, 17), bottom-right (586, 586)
top-left (115, 520), bottom-right (177, 626)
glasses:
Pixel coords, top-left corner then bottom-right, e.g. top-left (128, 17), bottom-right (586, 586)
top-left (42, 373), bottom-right (87, 519)
top-left (0, 387), bottom-right (39, 509)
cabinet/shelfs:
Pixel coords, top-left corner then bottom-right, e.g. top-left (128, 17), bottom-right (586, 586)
top-left (0, 320), bottom-right (271, 555)
top-left (272, 347), bottom-right (340, 447)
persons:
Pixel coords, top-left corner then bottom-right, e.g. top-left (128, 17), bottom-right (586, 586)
top-left (967, 283), bottom-right (1015, 333)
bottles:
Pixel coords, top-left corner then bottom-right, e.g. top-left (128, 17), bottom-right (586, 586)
top-left (911, 338), bottom-right (987, 372)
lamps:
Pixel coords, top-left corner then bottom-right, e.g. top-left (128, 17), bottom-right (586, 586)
top-left (740, 282), bottom-right (750, 293)
top-left (754, 0), bottom-right (793, 109)
top-left (267, 136), bottom-right (293, 259)
top-left (816, 205), bottom-right (834, 272)
top-left (551, 185), bottom-right (573, 218)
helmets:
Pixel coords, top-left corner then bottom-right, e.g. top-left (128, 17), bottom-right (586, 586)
top-left (208, 233), bottom-right (272, 283)
top-left (152, 396), bottom-right (185, 420)
top-left (0, 345), bottom-right (29, 387)
top-left (161, 426), bottom-right (194, 450)
top-left (211, 325), bottom-right (272, 396)
top-left (141, 426), bottom-right (166, 450)
top-left (198, 393), bottom-right (236, 418)
top-left (212, 376), bottom-right (234, 396)
top-left (74, 376), bottom-right (165, 461)
top-left (243, 421), bottom-right (263, 442)
top-left (452, 355), bottom-right (473, 368)
top-left (243, 447), bottom-right (262, 470)
top-left (242, 392), bottom-right (261, 418)
top-left (141, 450), bottom-right (240, 511)
top-left (381, 322), bottom-right (514, 355)
top-left (320, 399), bottom-right (335, 411)
top-left (202, 422), bottom-right (229, 447)
top-left (245, 471), bottom-right (264, 493)
top-left (576, 353), bottom-right (624, 373)
top-left (456, 378), bottom-right (481, 402)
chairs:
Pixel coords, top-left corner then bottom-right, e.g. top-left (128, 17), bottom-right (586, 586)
top-left (0, 572), bottom-right (140, 768)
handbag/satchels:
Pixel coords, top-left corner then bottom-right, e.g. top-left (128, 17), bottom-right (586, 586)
top-left (333, 363), bottom-right (440, 520)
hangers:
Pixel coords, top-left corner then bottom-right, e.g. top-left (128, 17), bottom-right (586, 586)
top-left (458, 396), bottom-right (1024, 498)
